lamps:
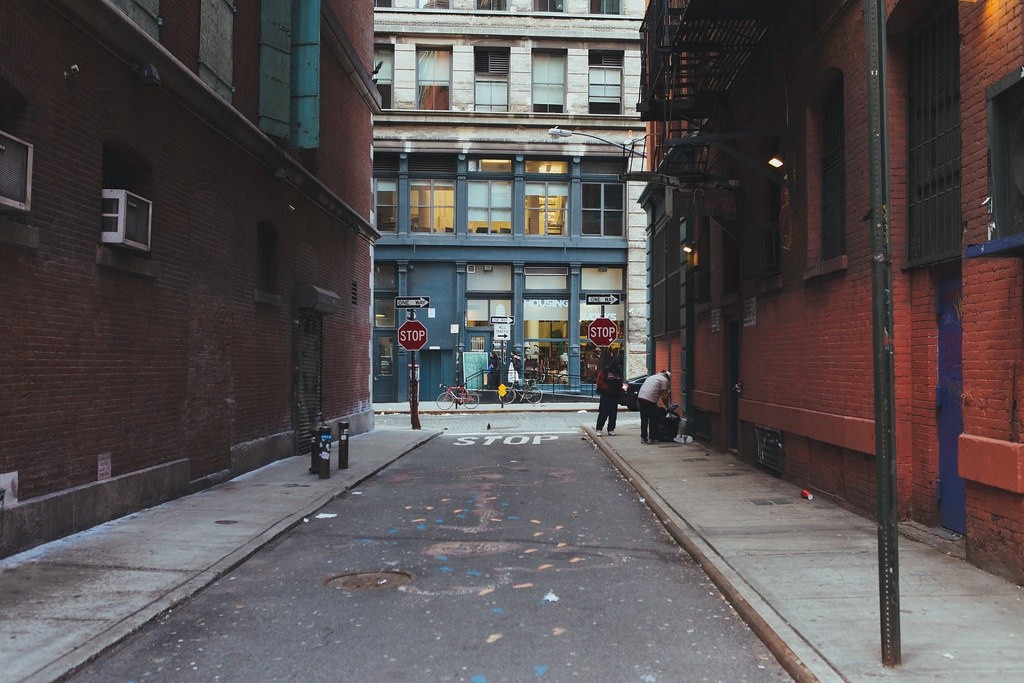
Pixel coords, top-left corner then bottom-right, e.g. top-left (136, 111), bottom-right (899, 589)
top-left (683, 240), bottom-right (699, 253)
top-left (768, 152), bottom-right (792, 182)
top-left (130, 62), bottom-right (162, 86)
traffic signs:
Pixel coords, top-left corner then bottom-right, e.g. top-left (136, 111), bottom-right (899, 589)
top-left (491, 316), bottom-right (515, 325)
top-left (394, 296), bottom-right (430, 310)
top-left (494, 324), bottom-right (511, 341)
top-left (585, 294), bottom-right (620, 305)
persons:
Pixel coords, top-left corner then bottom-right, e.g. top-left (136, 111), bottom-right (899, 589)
top-left (488, 352), bottom-right (495, 365)
top-left (636, 370), bottom-right (676, 445)
top-left (509, 349), bottom-right (525, 389)
top-left (489, 354), bottom-right (502, 386)
top-left (593, 354), bottom-right (626, 436)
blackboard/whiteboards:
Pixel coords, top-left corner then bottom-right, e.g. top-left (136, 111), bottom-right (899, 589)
top-left (463, 351), bottom-right (489, 390)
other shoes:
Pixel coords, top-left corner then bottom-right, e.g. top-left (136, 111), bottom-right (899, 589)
top-left (608, 431), bottom-right (616, 436)
top-left (641, 437), bottom-right (648, 443)
top-left (647, 439), bottom-right (655, 444)
top-left (597, 430), bottom-right (602, 436)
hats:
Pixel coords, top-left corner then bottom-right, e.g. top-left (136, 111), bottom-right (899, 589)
top-left (664, 370), bottom-right (672, 381)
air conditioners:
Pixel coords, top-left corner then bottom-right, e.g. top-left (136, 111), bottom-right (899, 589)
top-left (102, 188), bottom-right (152, 253)
top-left (0, 131), bottom-right (34, 213)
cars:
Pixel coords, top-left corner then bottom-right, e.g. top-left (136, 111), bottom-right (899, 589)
top-left (616, 374), bottom-right (648, 411)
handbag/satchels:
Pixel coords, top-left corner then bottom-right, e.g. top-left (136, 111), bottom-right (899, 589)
top-left (617, 388), bottom-right (625, 403)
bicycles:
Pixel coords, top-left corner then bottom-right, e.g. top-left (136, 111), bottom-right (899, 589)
top-left (499, 376), bottom-right (543, 405)
top-left (436, 382), bottom-right (480, 409)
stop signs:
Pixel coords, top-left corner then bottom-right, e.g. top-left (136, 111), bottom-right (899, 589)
top-left (398, 322), bottom-right (428, 351)
top-left (589, 317), bottom-right (618, 347)
